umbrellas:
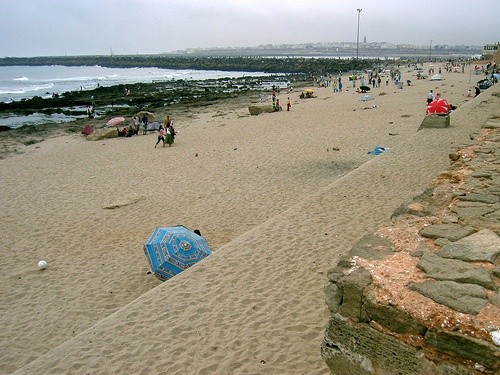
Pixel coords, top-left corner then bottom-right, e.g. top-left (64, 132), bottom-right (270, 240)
top-left (107, 116), bottom-right (125, 126)
top-left (359, 86), bottom-right (370, 91)
top-left (426, 98), bottom-right (449, 116)
top-left (137, 111), bottom-right (155, 120)
top-left (305, 89), bottom-right (315, 93)
top-left (143, 224), bottom-right (211, 281)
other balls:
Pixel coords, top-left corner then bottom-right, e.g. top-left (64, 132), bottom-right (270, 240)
top-left (38, 260), bottom-right (47, 269)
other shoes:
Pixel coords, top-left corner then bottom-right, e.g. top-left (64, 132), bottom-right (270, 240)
top-left (145, 133), bottom-right (146, 135)
top-left (155, 146), bottom-right (156, 148)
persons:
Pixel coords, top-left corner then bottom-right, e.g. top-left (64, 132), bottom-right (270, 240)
top-left (271, 84), bottom-right (316, 112)
top-left (317, 59), bottom-right (500, 115)
top-left (86, 89), bottom-right (175, 149)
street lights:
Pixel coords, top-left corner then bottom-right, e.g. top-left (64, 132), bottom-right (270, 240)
top-left (355, 8), bottom-right (363, 60)
top-left (429, 39), bottom-right (433, 63)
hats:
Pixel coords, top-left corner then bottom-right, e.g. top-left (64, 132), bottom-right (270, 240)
top-left (430, 90), bottom-right (433, 93)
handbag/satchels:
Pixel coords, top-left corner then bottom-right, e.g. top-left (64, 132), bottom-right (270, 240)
top-left (167, 128), bottom-right (172, 135)
top-left (158, 135), bottom-right (162, 138)
top-left (166, 122), bottom-right (170, 127)
top-left (140, 126), bottom-right (145, 130)
